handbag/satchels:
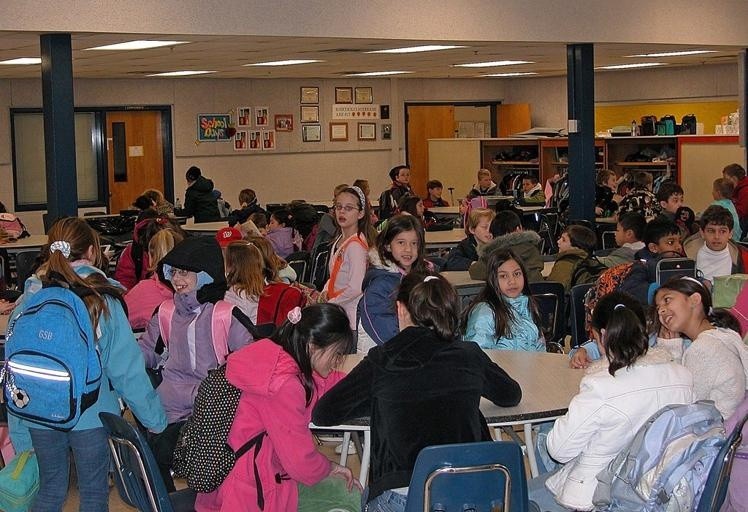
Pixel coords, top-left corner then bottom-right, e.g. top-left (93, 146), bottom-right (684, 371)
top-left (0, 449), bottom-right (41, 512)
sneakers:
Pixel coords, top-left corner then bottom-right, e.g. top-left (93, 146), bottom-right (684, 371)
top-left (336, 440), bottom-right (359, 455)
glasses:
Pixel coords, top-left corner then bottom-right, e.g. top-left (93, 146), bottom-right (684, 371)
top-left (335, 204), bottom-right (360, 212)
top-left (167, 268), bottom-right (188, 276)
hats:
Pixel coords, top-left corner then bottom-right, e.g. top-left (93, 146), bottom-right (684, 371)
top-left (185, 166), bottom-right (201, 181)
top-left (215, 227), bottom-right (243, 250)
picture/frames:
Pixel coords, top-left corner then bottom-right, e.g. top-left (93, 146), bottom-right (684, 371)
top-left (334, 87), bottom-right (353, 104)
top-left (300, 86), bottom-right (320, 104)
top-left (329, 122), bottom-right (348, 141)
top-left (357, 122), bottom-right (377, 141)
top-left (300, 105), bottom-right (319, 123)
top-left (302, 124), bottom-right (321, 142)
top-left (355, 87), bottom-right (373, 104)
top-left (197, 113), bottom-right (233, 143)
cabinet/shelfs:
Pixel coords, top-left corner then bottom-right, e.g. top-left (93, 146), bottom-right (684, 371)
top-left (480, 136), bottom-right (681, 204)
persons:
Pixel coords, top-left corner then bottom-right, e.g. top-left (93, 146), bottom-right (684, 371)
top-left (1, 164), bottom-right (748, 511)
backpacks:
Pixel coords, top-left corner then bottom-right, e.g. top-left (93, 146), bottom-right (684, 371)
top-left (582, 261), bottom-right (642, 339)
top-left (592, 400), bottom-right (728, 512)
top-left (170, 362), bottom-right (243, 493)
top-left (1, 286), bottom-right (103, 432)
top-left (253, 282), bottom-right (308, 342)
top-left (568, 255), bottom-right (610, 294)
